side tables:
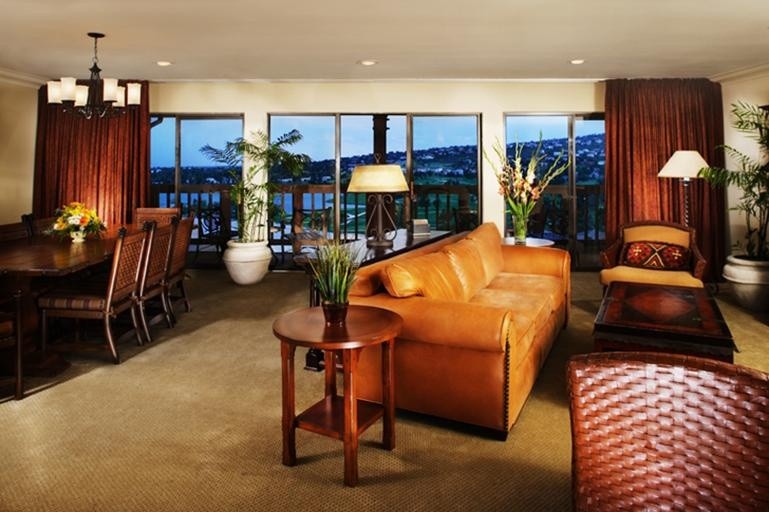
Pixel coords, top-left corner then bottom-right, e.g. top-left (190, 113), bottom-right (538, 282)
top-left (271, 306), bottom-right (403, 485)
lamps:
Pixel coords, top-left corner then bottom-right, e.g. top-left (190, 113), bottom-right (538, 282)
top-left (658, 150), bottom-right (715, 226)
top-left (346, 164), bottom-right (409, 256)
top-left (47, 32), bottom-right (142, 120)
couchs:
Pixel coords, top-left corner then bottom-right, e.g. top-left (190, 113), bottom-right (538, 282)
top-left (348, 222), bottom-right (571, 438)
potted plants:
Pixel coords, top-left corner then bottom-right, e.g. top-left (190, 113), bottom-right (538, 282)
top-left (697, 99), bottom-right (769, 312)
top-left (299, 240), bottom-right (367, 323)
top-left (198, 129), bottom-right (313, 285)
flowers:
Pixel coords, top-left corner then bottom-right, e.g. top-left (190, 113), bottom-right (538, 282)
top-left (45, 202), bottom-right (106, 240)
top-left (482, 130), bottom-right (571, 244)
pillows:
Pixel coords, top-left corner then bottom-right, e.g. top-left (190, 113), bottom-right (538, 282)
top-left (624, 241), bottom-right (693, 271)
top-left (380, 223), bottom-right (505, 303)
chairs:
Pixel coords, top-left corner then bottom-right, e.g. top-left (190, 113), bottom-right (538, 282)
top-left (565, 352), bottom-right (769, 511)
top-left (0, 208), bottom-right (194, 400)
top-left (599, 220), bottom-right (707, 287)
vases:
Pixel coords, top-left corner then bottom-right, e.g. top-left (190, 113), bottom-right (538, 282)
top-left (512, 216), bottom-right (529, 246)
